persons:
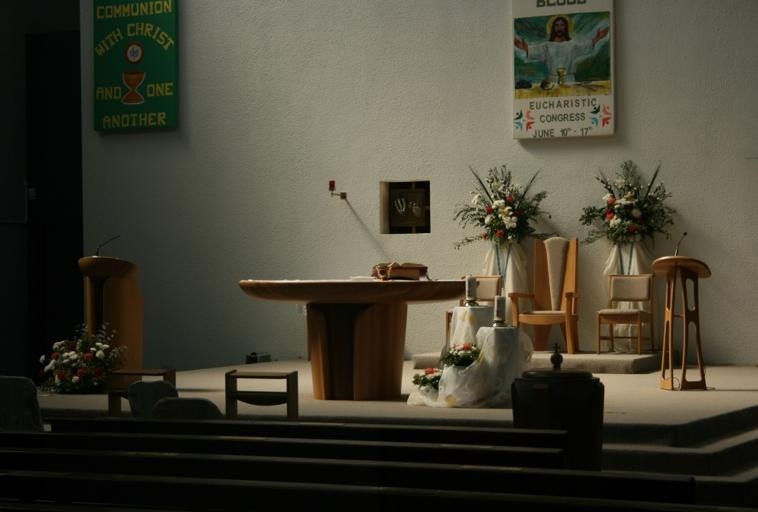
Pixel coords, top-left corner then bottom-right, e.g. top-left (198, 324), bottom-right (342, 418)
top-left (513, 14), bottom-right (609, 85)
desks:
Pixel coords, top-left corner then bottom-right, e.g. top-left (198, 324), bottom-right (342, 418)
top-left (239, 277), bottom-right (479, 402)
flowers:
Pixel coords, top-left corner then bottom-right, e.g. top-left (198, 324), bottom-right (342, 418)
top-left (411, 367), bottom-right (443, 392)
top-left (439, 343), bottom-right (481, 369)
top-left (577, 159), bottom-right (677, 254)
top-left (37, 321), bottom-right (128, 395)
top-left (450, 162), bottom-right (559, 251)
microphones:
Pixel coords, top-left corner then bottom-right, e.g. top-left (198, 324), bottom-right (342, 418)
top-left (673, 231), bottom-right (688, 256)
top-left (94, 233), bottom-right (121, 255)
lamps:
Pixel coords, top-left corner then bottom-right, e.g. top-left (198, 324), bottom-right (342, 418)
top-left (327, 180), bottom-right (346, 200)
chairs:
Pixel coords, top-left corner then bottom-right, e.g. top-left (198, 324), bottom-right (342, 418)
top-left (595, 272), bottom-right (655, 356)
top-left (444, 275), bottom-right (502, 343)
top-left (0, 375), bottom-right (44, 433)
top-left (506, 237), bottom-right (584, 354)
top-left (126, 380), bottom-right (221, 419)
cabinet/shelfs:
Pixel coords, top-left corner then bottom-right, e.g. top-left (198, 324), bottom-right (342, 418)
top-left (108, 368), bottom-right (175, 416)
top-left (224, 369), bottom-right (297, 419)
